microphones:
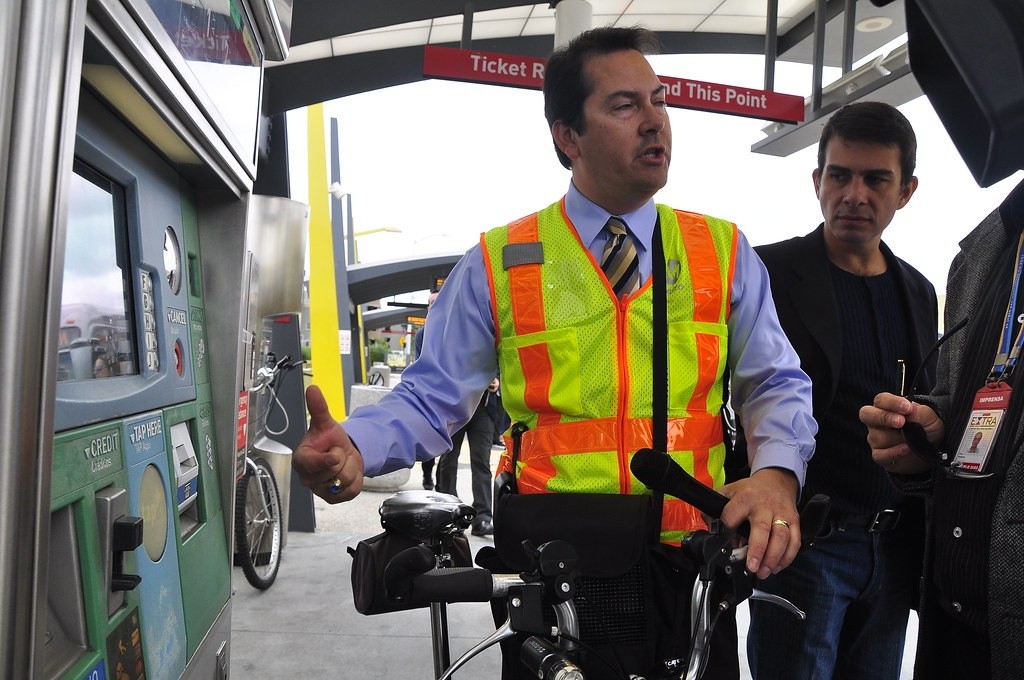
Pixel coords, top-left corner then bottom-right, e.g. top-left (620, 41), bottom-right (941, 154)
top-left (630, 448), bottom-right (752, 540)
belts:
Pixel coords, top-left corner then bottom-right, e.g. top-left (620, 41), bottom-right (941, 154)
top-left (829, 507), bottom-right (915, 532)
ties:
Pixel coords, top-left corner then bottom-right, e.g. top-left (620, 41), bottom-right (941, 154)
top-left (599, 216), bottom-right (641, 299)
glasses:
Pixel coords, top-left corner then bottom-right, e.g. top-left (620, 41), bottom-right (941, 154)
top-left (900, 316), bottom-right (1024, 478)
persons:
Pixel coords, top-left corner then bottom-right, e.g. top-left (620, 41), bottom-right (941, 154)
top-left (94, 355), bottom-right (120, 377)
top-left (857, 178), bottom-right (1024, 680)
top-left (290, 28), bottom-right (819, 680)
top-left (969, 432), bottom-right (982, 453)
top-left (732, 100), bottom-right (939, 680)
top-left (413, 290), bottom-right (510, 536)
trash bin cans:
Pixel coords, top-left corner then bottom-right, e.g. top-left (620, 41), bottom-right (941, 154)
top-left (367, 365), bottom-right (390, 386)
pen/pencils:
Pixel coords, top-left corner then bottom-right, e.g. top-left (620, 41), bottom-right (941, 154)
top-left (898, 359), bottom-right (907, 398)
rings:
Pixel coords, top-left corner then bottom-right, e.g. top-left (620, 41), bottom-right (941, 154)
top-left (328, 478), bottom-right (345, 493)
top-left (773, 520), bottom-right (790, 529)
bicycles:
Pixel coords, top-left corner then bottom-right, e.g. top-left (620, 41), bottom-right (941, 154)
top-left (234, 352), bottom-right (306, 594)
top-left (377, 446), bottom-right (806, 680)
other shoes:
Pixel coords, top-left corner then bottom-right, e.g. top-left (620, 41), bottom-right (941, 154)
top-left (424, 475), bottom-right (435, 490)
top-left (471, 523), bottom-right (493, 536)
